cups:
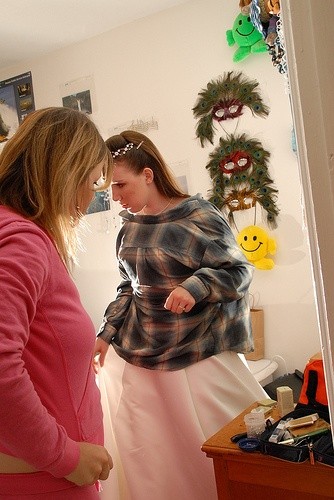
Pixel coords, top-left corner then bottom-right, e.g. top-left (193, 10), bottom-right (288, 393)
top-left (244, 413), bottom-right (265, 438)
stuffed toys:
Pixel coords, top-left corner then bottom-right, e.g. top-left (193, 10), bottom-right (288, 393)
top-left (235, 225), bottom-right (278, 271)
top-left (225, 0), bottom-right (282, 64)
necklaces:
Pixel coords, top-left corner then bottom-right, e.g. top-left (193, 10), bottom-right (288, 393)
top-left (142, 196), bottom-right (174, 216)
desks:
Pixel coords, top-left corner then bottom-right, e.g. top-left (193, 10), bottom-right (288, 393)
top-left (200, 400), bottom-right (333, 499)
top-left (247, 359), bottom-right (279, 385)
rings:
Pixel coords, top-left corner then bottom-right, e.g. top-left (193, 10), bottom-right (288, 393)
top-left (178, 305), bottom-right (186, 311)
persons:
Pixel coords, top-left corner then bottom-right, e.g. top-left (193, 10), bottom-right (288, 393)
top-left (90, 130), bottom-right (272, 500)
top-left (0, 106), bottom-right (116, 500)
